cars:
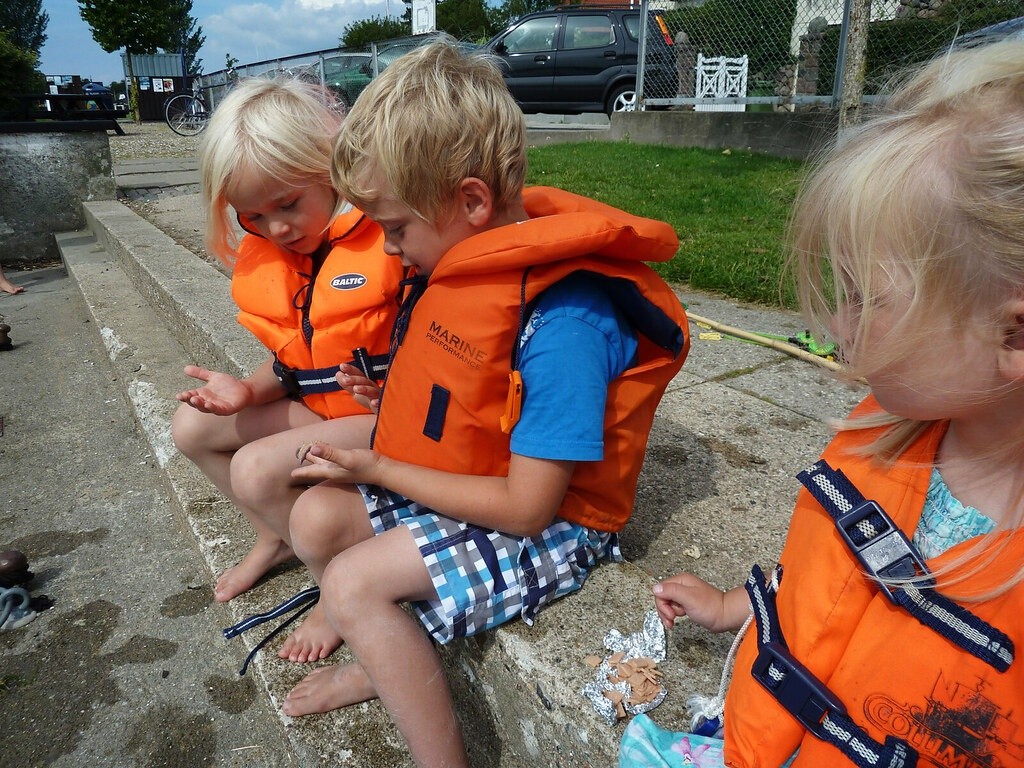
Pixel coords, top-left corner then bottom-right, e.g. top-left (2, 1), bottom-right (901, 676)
top-left (325, 39), bottom-right (489, 115)
top-left (950, 16), bottom-right (1024, 72)
top-left (250, 51), bottom-right (380, 110)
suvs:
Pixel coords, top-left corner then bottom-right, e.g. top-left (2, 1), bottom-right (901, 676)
top-left (453, 3), bottom-right (678, 126)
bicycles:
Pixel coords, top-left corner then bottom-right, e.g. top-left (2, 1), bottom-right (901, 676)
top-left (162, 67), bottom-right (242, 137)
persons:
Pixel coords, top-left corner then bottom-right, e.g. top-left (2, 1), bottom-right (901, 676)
top-left (285, 30), bottom-right (693, 767)
top-left (172, 70), bottom-right (413, 665)
top-left (653, 19), bottom-right (1024, 768)
